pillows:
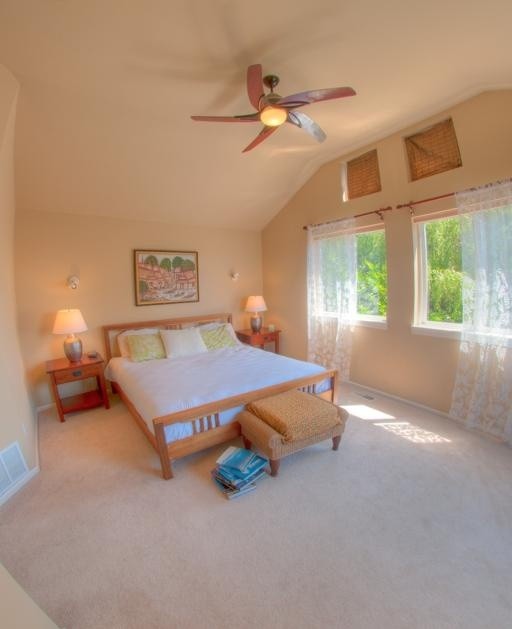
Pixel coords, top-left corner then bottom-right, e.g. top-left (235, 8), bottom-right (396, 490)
top-left (127, 334), bottom-right (164, 359)
top-left (200, 325), bottom-right (235, 350)
top-left (195, 324), bottom-right (239, 351)
top-left (160, 328), bottom-right (207, 357)
top-left (116, 328), bottom-right (160, 358)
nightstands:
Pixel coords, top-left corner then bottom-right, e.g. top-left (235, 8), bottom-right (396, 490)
top-left (45, 353), bottom-right (110, 422)
top-left (236, 327), bottom-right (282, 355)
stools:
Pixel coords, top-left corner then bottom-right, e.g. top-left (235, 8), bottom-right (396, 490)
top-left (238, 388), bottom-right (349, 477)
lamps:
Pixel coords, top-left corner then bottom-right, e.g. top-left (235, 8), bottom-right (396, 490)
top-left (260, 105), bottom-right (288, 126)
top-left (52, 309), bottom-right (89, 362)
top-left (245, 296), bottom-right (267, 332)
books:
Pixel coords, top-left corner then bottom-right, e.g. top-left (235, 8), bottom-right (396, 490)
top-left (210, 446), bottom-right (268, 500)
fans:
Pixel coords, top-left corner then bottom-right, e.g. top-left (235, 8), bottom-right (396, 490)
top-left (192, 64), bottom-right (356, 154)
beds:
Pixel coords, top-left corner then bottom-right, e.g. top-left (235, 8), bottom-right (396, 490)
top-left (104, 313), bottom-right (338, 480)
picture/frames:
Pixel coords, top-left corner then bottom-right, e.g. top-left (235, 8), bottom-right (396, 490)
top-left (133, 249), bottom-right (200, 307)
